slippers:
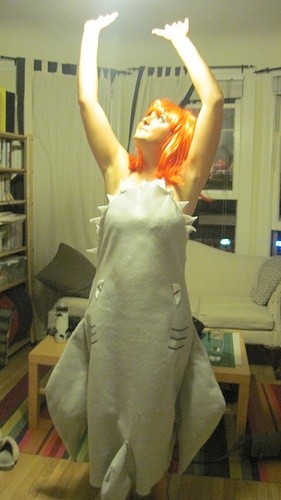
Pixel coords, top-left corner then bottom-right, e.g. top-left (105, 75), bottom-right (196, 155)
top-left (0, 436), bottom-right (20, 470)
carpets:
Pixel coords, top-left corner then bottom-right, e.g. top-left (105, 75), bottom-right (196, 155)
top-left (0, 364), bottom-right (281, 483)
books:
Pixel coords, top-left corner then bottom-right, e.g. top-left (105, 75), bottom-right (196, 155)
top-left (0, 136), bottom-right (29, 286)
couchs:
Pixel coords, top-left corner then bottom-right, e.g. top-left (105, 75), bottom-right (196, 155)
top-left (47, 239), bottom-right (281, 348)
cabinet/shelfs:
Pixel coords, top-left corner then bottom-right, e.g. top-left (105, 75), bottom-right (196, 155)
top-left (0, 132), bottom-right (35, 367)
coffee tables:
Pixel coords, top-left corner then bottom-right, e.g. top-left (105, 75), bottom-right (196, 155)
top-left (28, 329), bottom-right (251, 447)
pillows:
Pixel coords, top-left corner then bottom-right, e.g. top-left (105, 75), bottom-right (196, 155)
top-left (35, 242), bottom-right (97, 299)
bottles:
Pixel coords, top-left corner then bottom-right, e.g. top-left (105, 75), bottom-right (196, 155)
top-left (55, 302), bottom-right (69, 343)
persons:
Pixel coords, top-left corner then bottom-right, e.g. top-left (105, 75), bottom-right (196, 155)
top-left (44, 10), bottom-right (225, 500)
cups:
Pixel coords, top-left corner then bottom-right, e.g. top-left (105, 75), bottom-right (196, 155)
top-left (208, 329), bottom-right (224, 358)
